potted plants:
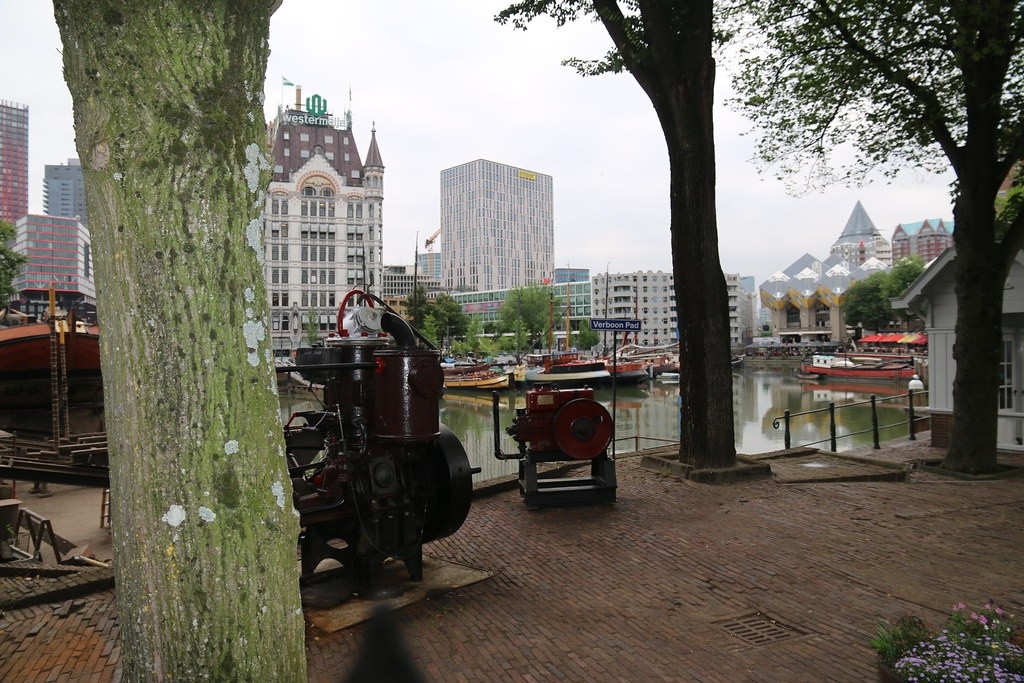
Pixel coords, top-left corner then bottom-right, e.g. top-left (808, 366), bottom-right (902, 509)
top-left (869, 612), bottom-right (931, 682)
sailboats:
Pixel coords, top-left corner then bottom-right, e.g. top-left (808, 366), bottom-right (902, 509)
top-left (439, 271), bottom-right (680, 388)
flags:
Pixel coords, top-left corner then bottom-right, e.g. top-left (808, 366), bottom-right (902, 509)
top-left (282, 77), bottom-right (295, 86)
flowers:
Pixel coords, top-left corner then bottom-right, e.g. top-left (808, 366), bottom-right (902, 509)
top-left (895, 598), bottom-right (1024, 683)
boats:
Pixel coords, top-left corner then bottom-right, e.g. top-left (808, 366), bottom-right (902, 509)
top-left (731, 353), bottom-right (746, 368)
top-left (791, 350), bottom-right (917, 380)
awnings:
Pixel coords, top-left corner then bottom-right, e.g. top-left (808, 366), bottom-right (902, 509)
top-left (857, 331), bottom-right (928, 346)
